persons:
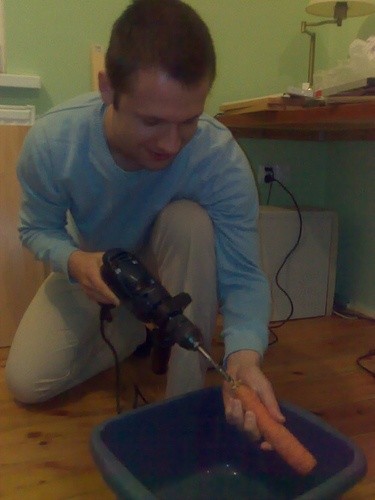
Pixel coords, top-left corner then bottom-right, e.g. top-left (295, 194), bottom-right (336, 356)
top-left (6, 1), bottom-right (287, 450)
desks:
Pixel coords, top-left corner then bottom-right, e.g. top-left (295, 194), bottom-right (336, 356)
top-left (215, 104), bottom-right (375, 139)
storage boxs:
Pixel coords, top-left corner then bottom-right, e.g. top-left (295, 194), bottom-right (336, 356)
top-left (257, 204), bottom-right (337, 322)
top-left (90, 384), bottom-right (368, 500)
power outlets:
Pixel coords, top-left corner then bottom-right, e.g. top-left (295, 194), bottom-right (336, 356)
top-left (258, 164), bottom-right (280, 185)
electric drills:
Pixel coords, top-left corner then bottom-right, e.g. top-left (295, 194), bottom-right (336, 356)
top-left (99, 248), bottom-right (234, 387)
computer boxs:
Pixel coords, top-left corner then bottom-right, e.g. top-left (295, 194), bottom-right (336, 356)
top-left (255, 204), bottom-right (339, 322)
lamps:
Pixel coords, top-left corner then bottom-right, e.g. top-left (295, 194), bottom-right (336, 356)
top-left (287, 0), bottom-right (375, 99)
